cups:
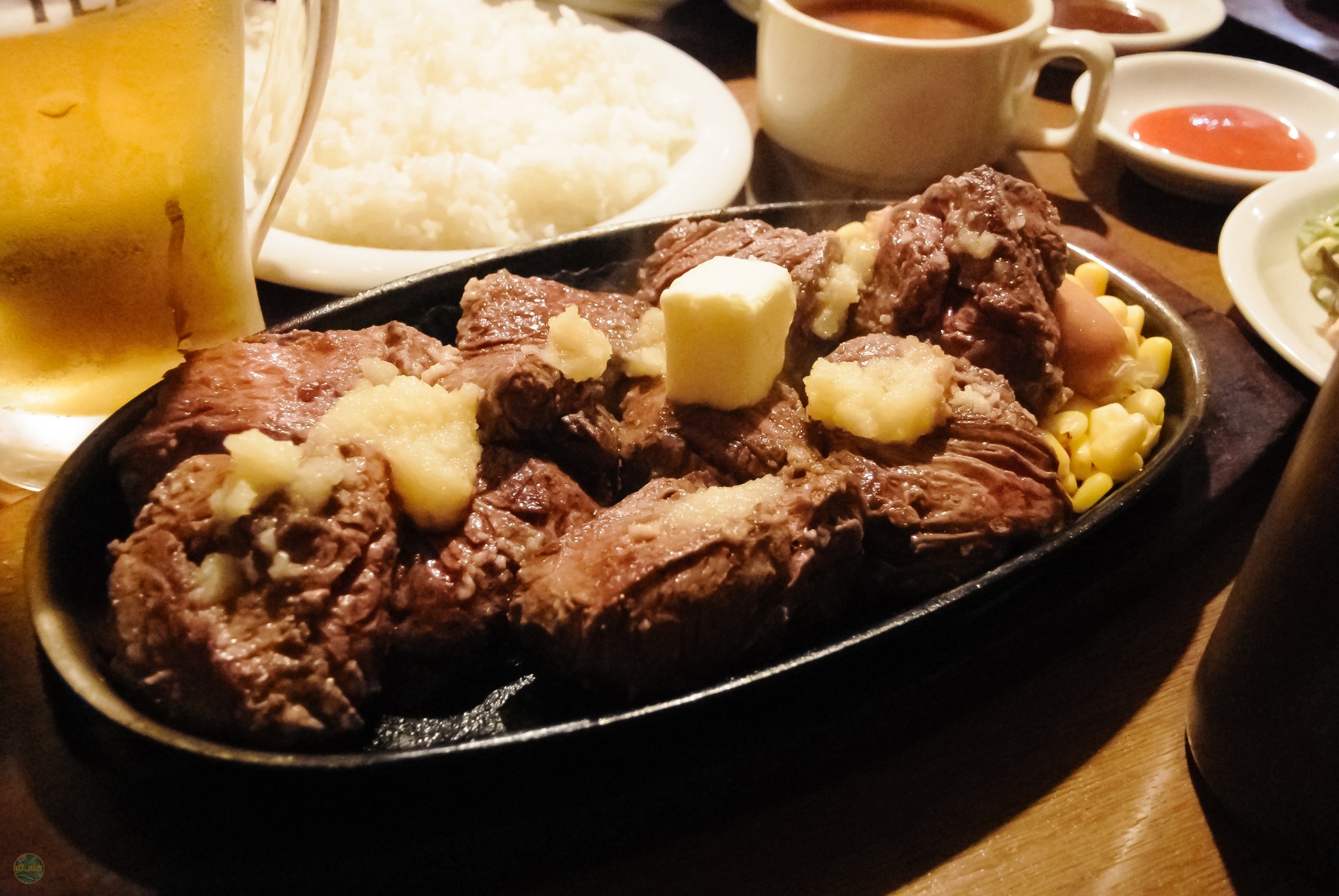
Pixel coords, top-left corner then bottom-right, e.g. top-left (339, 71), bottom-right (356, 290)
top-left (759, 0), bottom-right (1117, 198)
top-left (0, 0), bottom-right (339, 496)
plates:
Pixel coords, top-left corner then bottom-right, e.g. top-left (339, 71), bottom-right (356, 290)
top-left (1072, 49), bottom-right (1339, 202)
top-left (26, 198), bottom-right (1211, 771)
top-left (1042, 1), bottom-right (1228, 67)
top-left (243, 1), bottom-right (758, 300)
top-left (1217, 165), bottom-right (1339, 389)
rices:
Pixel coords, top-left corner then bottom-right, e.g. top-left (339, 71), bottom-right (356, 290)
top-left (241, 0), bottom-right (697, 249)
